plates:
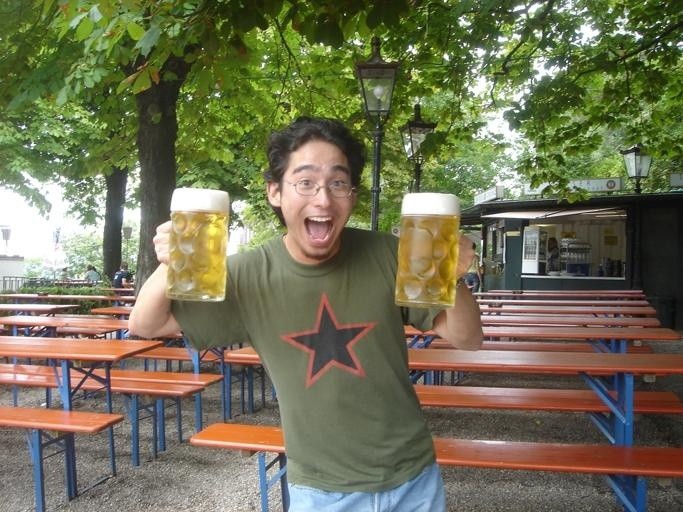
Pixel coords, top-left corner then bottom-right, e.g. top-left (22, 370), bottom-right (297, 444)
top-left (37, 292), bottom-right (49, 296)
top-left (592, 256), bottom-right (622, 277)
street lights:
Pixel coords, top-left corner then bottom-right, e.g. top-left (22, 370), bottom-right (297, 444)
top-left (620, 136), bottom-right (657, 290)
top-left (122, 224), bottom-right (133, 265)
top-left (353, 31), bottom-right (400, 236)
top-left (398, 103), bottom-right (438, 197)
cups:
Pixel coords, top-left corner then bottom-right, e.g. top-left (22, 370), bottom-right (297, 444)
top-left (164, 187), bottom-right (229, 302)
top-left (396, 195), bottom-right (463, 309)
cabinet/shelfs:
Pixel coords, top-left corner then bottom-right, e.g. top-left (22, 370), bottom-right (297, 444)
top-left (521, 227), bottom-right (548, 274)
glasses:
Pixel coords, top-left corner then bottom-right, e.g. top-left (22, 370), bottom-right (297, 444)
top-left (279, 177), bottom-right (356, 199)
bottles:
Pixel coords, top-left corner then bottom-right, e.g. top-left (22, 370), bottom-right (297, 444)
top-left (559, 239), bottom-right (592, 264)
top-left (525, 240), bottom-right (536, 259)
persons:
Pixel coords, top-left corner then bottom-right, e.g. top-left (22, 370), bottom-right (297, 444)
top-left (465, 243), bottom-right (484, 296)
top-left (128, 114), bottom-right (481, 512)
top-left (546, 239), bottom-right (561, 274)
top-left (112, 262), bottom-right (136, 299)
top-left (62, 262), bottom-right (103, 285)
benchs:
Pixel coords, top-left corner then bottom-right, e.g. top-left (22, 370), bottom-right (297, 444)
top-left (2, 288), bottom-right (288, 511)
top-left (404, 287), bottom-right (682, 510)
